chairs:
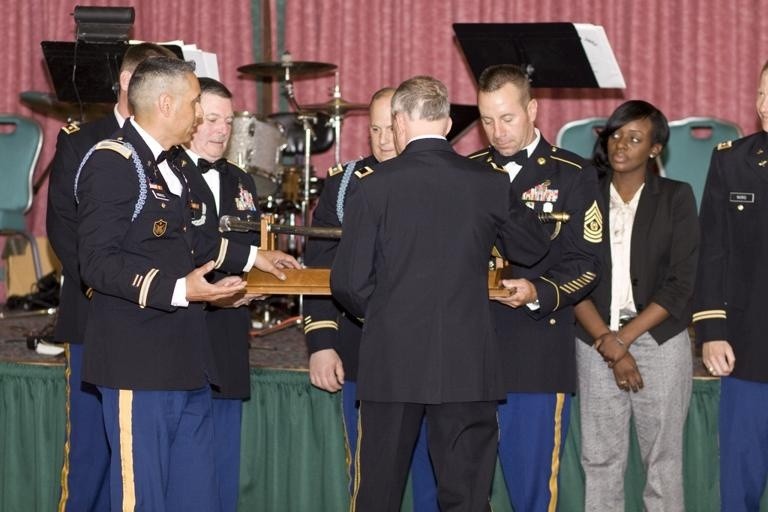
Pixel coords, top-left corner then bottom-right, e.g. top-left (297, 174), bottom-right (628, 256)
top-left (653, 117), bottom-right (744, 213)
top-left (0, 114), bottom-right (44, 290)
top-left (258, 113), bottom-right (335, 333)
top-left (556, 117), bottom-right (610, 159)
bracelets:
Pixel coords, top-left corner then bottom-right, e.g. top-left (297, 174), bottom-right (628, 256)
top-left (612, 329), bottom-right (626, 350)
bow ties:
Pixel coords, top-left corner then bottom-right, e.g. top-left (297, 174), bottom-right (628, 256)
top-left (493, 150), bottom-right (528, 167)
top-left (198, 158), bottom-right (228, 174)
top-left (157, 146), bottom-right (179, 165)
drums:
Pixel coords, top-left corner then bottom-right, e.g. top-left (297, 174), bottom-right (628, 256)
top-left (260, 166), bottom-right (303, 212)
top-left (226, 111), bottom-right (287, 199)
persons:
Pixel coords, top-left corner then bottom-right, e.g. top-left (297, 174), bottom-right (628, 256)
top-left (460, 63), bottom-right (602, 512)
top-left (74, 58), bottom-right (308, 512)
top-left (572, 96), bottom-right (700, 510)
top-left (302, 88), bottom-right (440, 509)
top-left (692, 59), bottom-right (768, 510)
top-left (46, 42), bottom-right (176, 512)
top-left (327, 73), bottom-right (553, 512)
top-left (164, 78), bottom-right (260, 512)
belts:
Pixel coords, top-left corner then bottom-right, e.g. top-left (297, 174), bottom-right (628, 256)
top-left (607, 314), bottom-right (636, 330)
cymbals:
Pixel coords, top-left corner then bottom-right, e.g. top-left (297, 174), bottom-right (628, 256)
top-left (301, 100), bottom-right (371, 110)
top-left (20, 91), bottom-right (113, 123)
top-left (236, 59), bottom-right (338, 76)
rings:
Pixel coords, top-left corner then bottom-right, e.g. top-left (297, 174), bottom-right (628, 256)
top-left (705, 366), bottom-right (716, 374)
top-left (618, 378), bottom-right (628, 385)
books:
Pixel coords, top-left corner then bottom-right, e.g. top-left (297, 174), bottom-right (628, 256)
top-left (488, 269), bottom-right (517, 298)
top-left (238, 268), bottom-right (331, 297)
top-left (573, 22), bottom-right (628, 91)
top-left (128, 39), bottom-right (219, 82)
